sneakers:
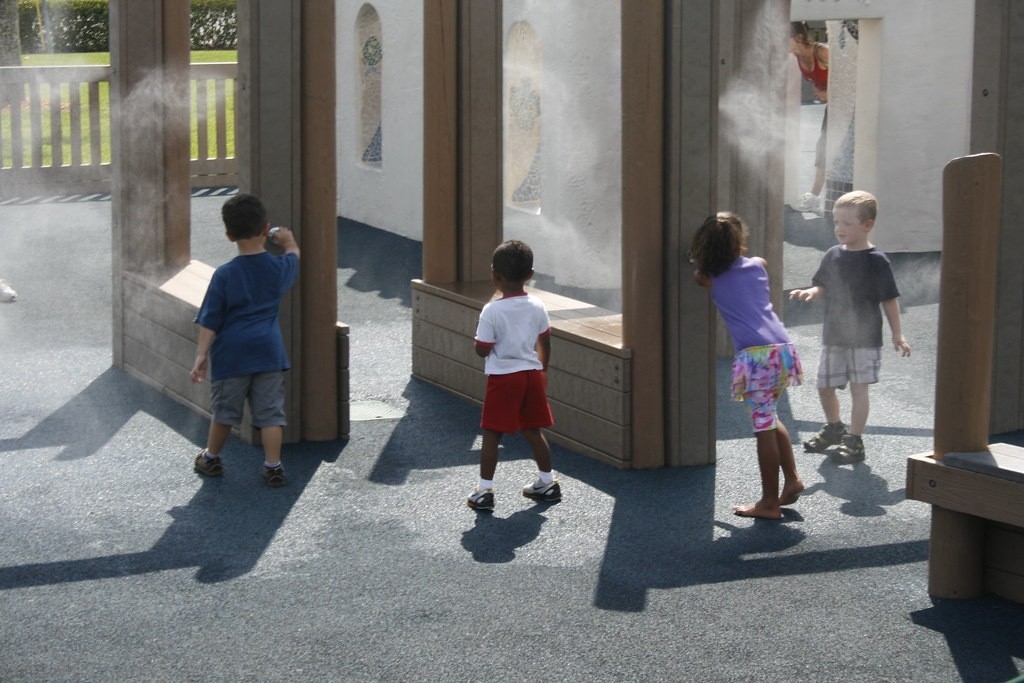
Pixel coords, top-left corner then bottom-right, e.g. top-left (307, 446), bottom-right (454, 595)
top-left (521, 478), bottom-right (562, 503)
top-left (466, 487), bottom-right (496, 511)
top-left (193, 448), bottom-right (224, 476)
top-left (260, 463), bottom-right (286, 487)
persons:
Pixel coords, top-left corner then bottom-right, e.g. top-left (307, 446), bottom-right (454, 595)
top-left (185, 194), bottom-right (303, 490)
top-left (686, 209), bottom-right (805, 522)
top-left (789, 22), bottom-right (831, 213)
top-left (788, 188), bottom-right (913, 462)
top-left (466, 239), bottom-right (564, 512)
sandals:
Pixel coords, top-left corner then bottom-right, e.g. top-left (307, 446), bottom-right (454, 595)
top-left (804, 420), bottom-right (866, 465)
